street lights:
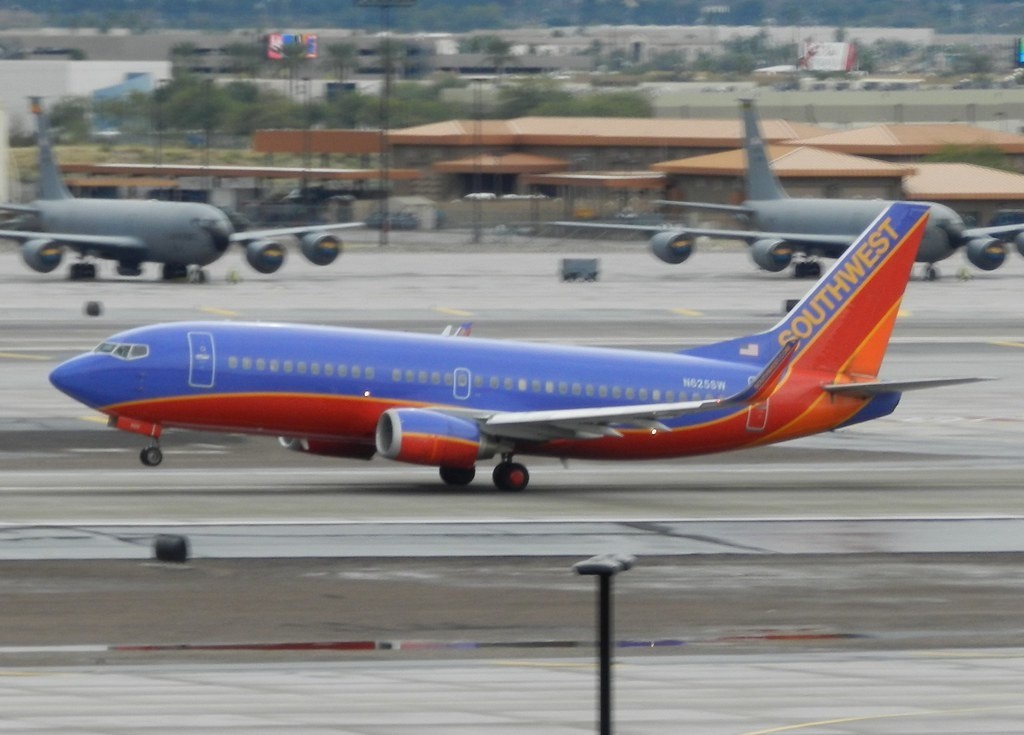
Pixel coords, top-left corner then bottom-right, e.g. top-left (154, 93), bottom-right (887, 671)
top-left (573, 552), bottom-right (639, 734)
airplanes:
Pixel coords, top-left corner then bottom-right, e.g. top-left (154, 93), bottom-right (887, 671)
top-left (1, 195), bottom-right (366, 285)
top-left (543, 96), bottom-right (1024, 281)
top-left (45, 202), bottom-right (1005, 495)
